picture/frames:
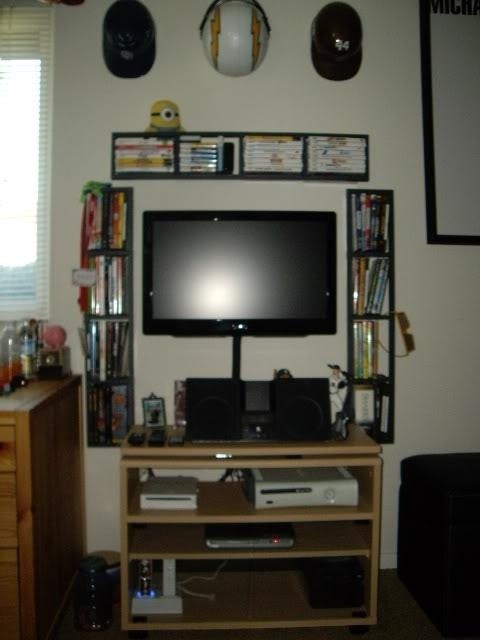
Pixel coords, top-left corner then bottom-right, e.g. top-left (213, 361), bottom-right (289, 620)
top-left (142, 398), bottom-right (166, 428)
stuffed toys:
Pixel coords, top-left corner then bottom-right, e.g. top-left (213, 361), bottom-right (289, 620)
top-left (145, 99), bottom-right (187, 144)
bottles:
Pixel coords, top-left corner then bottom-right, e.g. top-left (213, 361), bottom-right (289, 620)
top-left (0, 321), bottom-right (37, 389)
top-left (75, 549), bottom-right (120, 635)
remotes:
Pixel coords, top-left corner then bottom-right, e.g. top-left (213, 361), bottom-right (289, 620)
top-left (127, 431), bottom-right (146, 446)
top-left (148, 428), bottom-right (166, 446)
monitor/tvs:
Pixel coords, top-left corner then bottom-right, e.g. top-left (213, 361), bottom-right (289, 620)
top-left (143, 210), bottom-right (337, 338)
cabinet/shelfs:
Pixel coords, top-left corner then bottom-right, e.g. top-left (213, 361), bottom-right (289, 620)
top-left (81, 185), bottom-right (135, 448)
top-left (345, 188), bottom-right (397, 444)
top-left (110, 131), bottom-right (371, 181)
top-left (118, 420), bottom-right (384, 639)
top-left (398, 453), bottom-right (479, 637)
top-left (0, 374), bottom-right (86, 639)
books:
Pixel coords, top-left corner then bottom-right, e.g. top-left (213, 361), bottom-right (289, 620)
top-left (114, 131), bottom-right (369, 178)
top-left (86, 185), bottom-right (132, 445)
top-left (350, 190), bottom-right (394, 427)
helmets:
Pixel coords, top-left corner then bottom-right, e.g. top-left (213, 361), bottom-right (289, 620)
top-left (101, 1), bottom-right (156, 78)
top-left (309, 1), bottom-right (363, 81)
top-left (197, 0), bottom-right (271, 78)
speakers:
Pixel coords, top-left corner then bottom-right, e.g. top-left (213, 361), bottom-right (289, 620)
top-left (269, 377), bottom-right (330, 443)
top-left (182, 379), bottom-right (246, 439)
